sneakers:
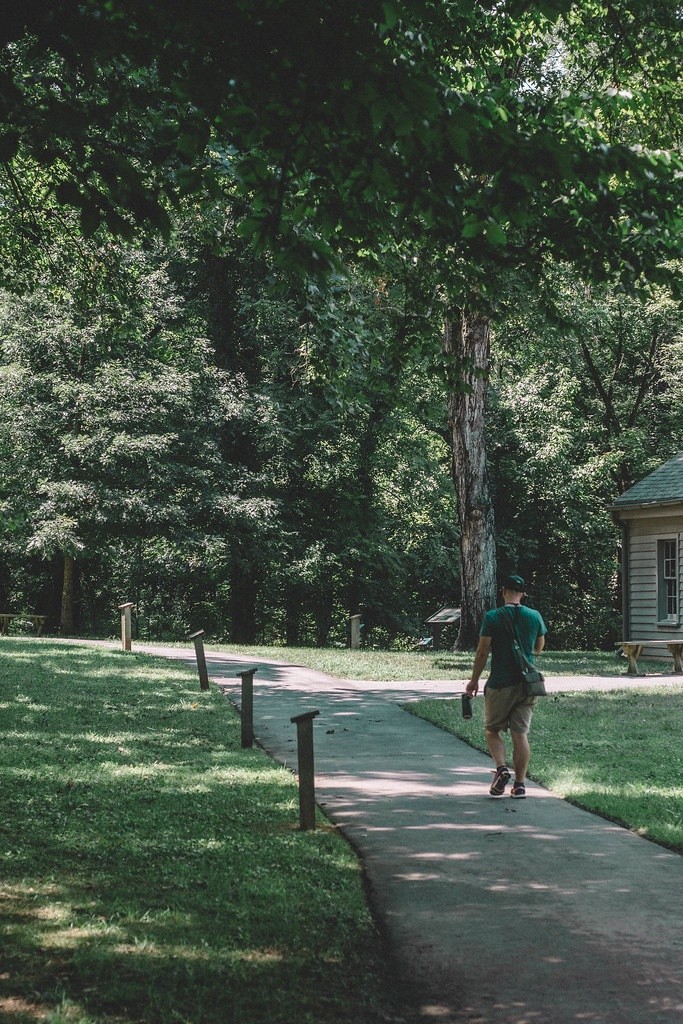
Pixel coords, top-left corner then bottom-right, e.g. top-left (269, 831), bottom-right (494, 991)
top-left (511, 786), bottom-right (527, 799)
top-left (490, 767), bottom-right (511, 795)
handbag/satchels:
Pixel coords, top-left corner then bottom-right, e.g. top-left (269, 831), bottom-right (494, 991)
top-left (522, 671), bottom-right (547, 696)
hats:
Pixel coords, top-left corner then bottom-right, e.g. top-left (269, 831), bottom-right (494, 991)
top-left (503, 575), bottom-right (528, 592)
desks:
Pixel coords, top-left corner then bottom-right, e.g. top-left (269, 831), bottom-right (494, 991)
top-left (613, 640), bottom-right (683, 675)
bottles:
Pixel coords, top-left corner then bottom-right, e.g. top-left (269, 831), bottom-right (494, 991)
top-left (462, 693), bottom-right (473, 720)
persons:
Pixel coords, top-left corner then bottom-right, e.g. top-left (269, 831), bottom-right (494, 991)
top-left (466, 576), bottom-right (548, 799)
